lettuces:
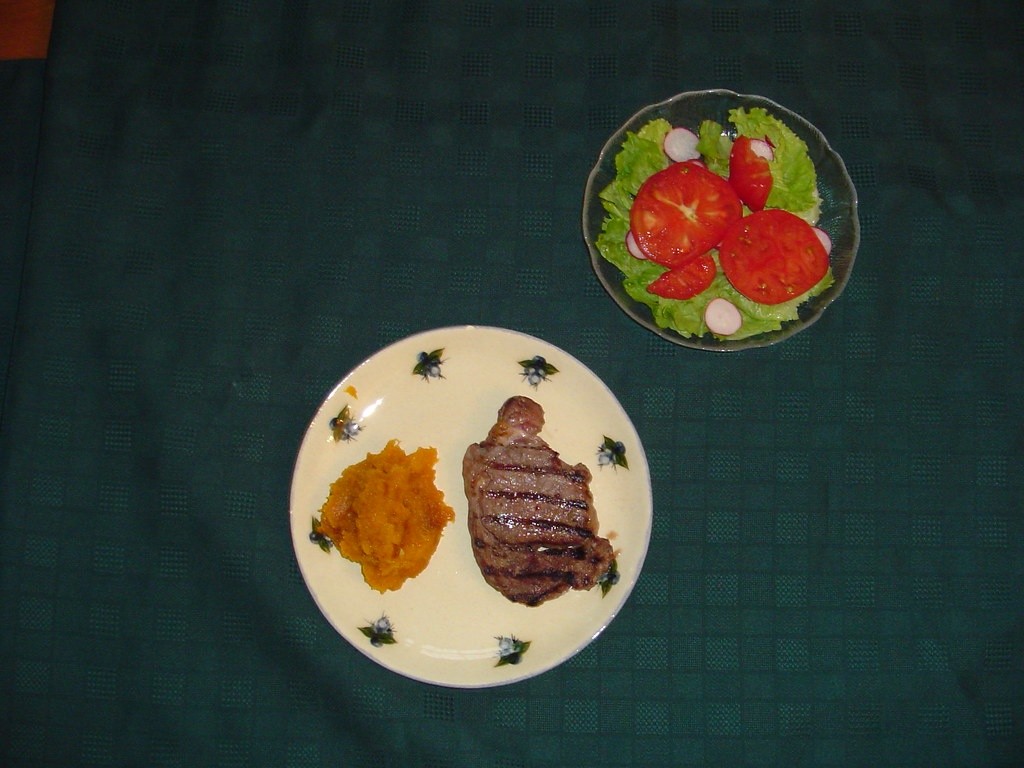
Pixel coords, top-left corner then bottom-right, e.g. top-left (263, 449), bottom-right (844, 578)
top-left (598, 105), bottom-right (835, 337)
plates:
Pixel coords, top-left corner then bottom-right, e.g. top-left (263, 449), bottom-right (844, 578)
top-left (289, 325), bottom-right (653, 688)
top-left (582, 89), bottom-right (861, 351)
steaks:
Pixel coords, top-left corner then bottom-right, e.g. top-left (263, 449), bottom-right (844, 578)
top-left (464, 394), bottom-right (611, 606)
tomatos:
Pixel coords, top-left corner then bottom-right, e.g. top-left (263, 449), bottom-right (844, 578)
top-left (720, 209), bottom-right (829, 304)
top-left (630, 163), bottom-right (742, 265)
top-left (647, 253), bottom-right (715, 297)
top-left (729, 134), bottom-right (773, 212)
